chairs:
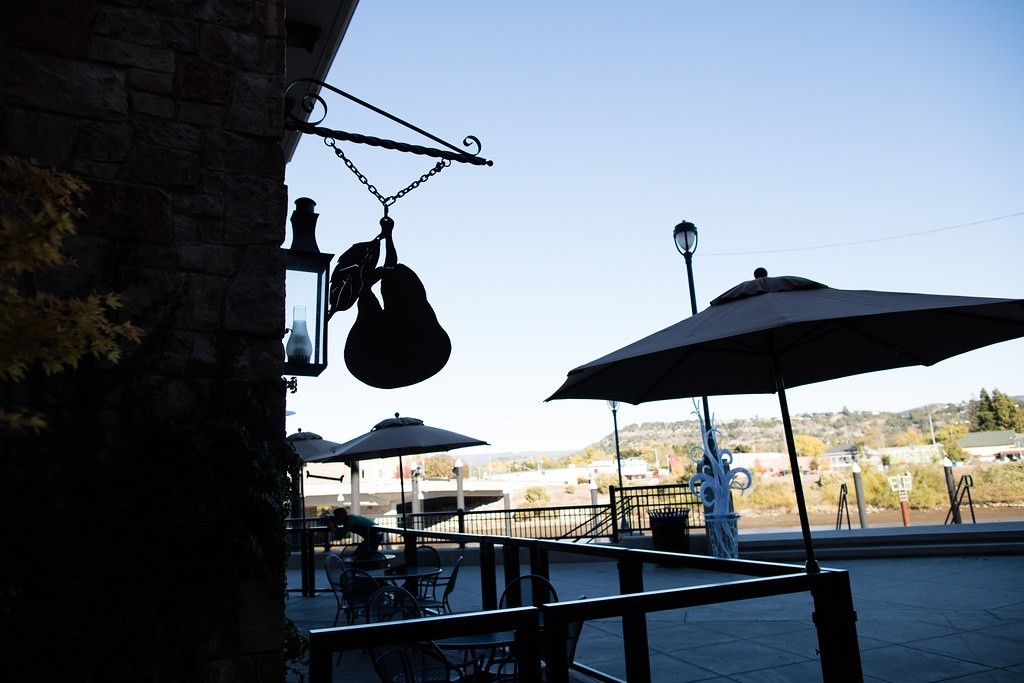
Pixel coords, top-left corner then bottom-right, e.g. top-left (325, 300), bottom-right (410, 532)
top-left (366, 584), bottom-right (422, 623)
top-left (370, 642), bottom-right (449, 683)
top-left (417, 554), bottom-right (464, 615)
top-left (355, 551), bottom-right (399, 608)
top-left (496, 574), bottom-right (561, 633)
top-left (338, 567), bottom-right (380, 625)
top-left (324, 553), bottom-right (349, 628)
top-left (402, 545), bottom-right (442, 614)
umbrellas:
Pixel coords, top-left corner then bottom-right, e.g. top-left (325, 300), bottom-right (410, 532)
top-left (543, 267), bottom-right (1024, 575)
top-left (302, 412), bottom-right (492, 570)
top-left (287, 428), bottom-right (342, 531)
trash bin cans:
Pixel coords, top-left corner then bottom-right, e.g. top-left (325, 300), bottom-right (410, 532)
top-left (646, 507), bottom-right (691, 553)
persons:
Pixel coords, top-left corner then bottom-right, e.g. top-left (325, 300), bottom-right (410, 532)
top-left (326, 507), bottom-right (385, 561)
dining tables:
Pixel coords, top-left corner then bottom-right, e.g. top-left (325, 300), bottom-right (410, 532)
top-left (432, 630), bottom-right (518, 683)
top-left (354, 566), bottom-right (444, 617)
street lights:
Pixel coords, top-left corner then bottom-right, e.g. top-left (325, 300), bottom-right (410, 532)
top-left (672, 218), bottom-right (740, 560)
top-left (337, 493), bottom-right (345, 545)
top-left (607, 400), bottom-right (629, 531)
top-left (454, 457), bottom-right (465, 534)
top-left (943, 456), bottom-right (962, 524)
top-left (410, 460), bottom-right (423, 542)
top-left (589, 479), bottom-right (599, 535)
top-left (502, 487), bottom-right (512, 537)
top-left (851, 462), bottom-right (868, 529)
